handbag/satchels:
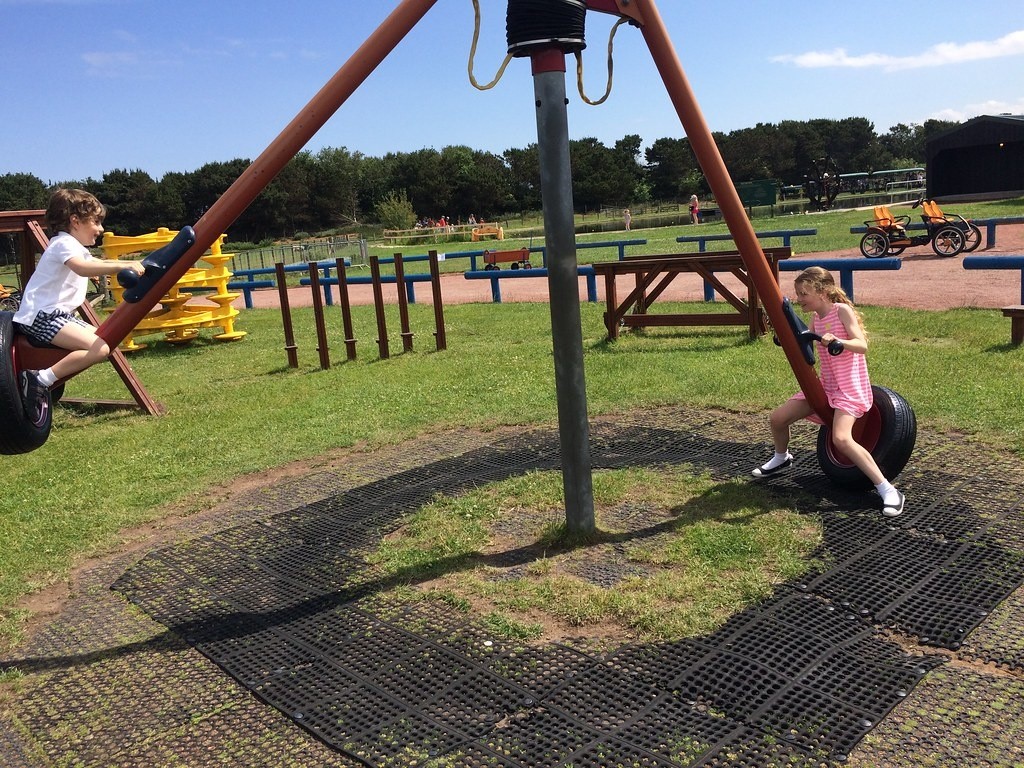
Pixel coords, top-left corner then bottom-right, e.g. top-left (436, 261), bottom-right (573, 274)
top-left (689, 206), bottom-right (694, 211)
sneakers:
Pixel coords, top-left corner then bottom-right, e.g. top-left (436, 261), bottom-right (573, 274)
top-left (21, 368), bottom-right (49, 427)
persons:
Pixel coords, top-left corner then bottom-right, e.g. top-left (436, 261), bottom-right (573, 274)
top-left (624, 209), bottom-right (631, 231)
top-left (751, 265), bottom-right (905, 516)
top-left (11, 188), bottom-right (145, 424)
top-left (690, 195), bottom-right (699, 225)
top-left (415, 213), bottom-right (484, 229)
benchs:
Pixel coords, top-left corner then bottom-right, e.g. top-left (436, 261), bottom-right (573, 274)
top-left (472, 226), bottom-right (504, 242)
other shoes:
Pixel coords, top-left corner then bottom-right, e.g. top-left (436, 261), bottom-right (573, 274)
top-left (882, 490), bottom-right (905, 516)
top-left (752, 454), bottom-right (792, 477)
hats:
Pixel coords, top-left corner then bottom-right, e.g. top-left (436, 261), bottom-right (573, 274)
top-left (690, 195), bottom-right (697, 200)
